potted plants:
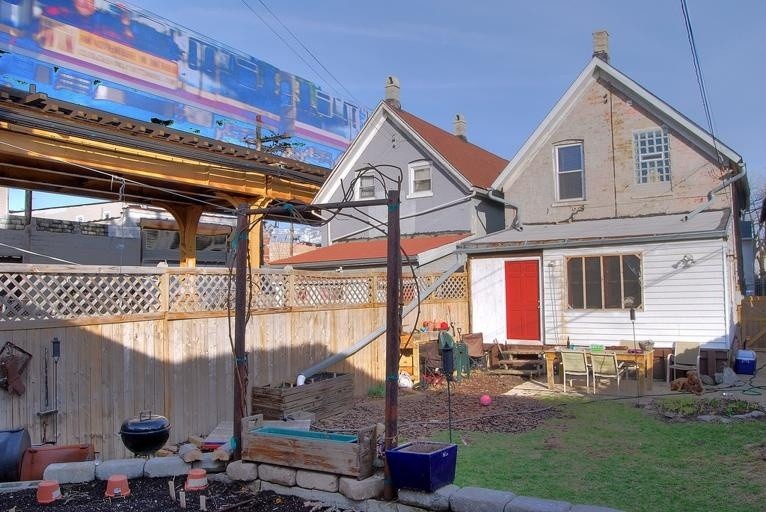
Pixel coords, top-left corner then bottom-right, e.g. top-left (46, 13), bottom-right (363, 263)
top-left (385, 442), bottom-right (458, 493)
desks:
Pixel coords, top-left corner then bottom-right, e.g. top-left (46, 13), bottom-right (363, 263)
top-left (544, 347), bottom-right (656, 396)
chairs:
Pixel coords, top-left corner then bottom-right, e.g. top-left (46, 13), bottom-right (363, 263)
top-left (561, 350), bottom-right (592, 394)
top-left (667, 341), bottom-right (701, 385)
top-left (440, 332), bottom-right (470, 386)
top-left (590, 352), bottom-right (629, 395)
top-left (462, 333), bottom-right (487, 373)
top-left (423, 342), bottom-right (444, 385)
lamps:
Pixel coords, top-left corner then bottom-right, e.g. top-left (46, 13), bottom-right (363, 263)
top-left (672, 256), bottom-right (696, 269)
top-left (630, 306), bottom-right (636, 322)
top-left (53, 338), bottom-right (60, 443)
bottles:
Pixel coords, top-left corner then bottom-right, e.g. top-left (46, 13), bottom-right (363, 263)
top-left (567, 336), bottom-right (570, 349)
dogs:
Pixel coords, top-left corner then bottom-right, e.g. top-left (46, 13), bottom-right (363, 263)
top-left (670, 373), bottom-right (705, 396)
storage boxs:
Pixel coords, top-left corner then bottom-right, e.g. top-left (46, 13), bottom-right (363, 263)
top-left (735, 350), bottom-right (757, 375)
